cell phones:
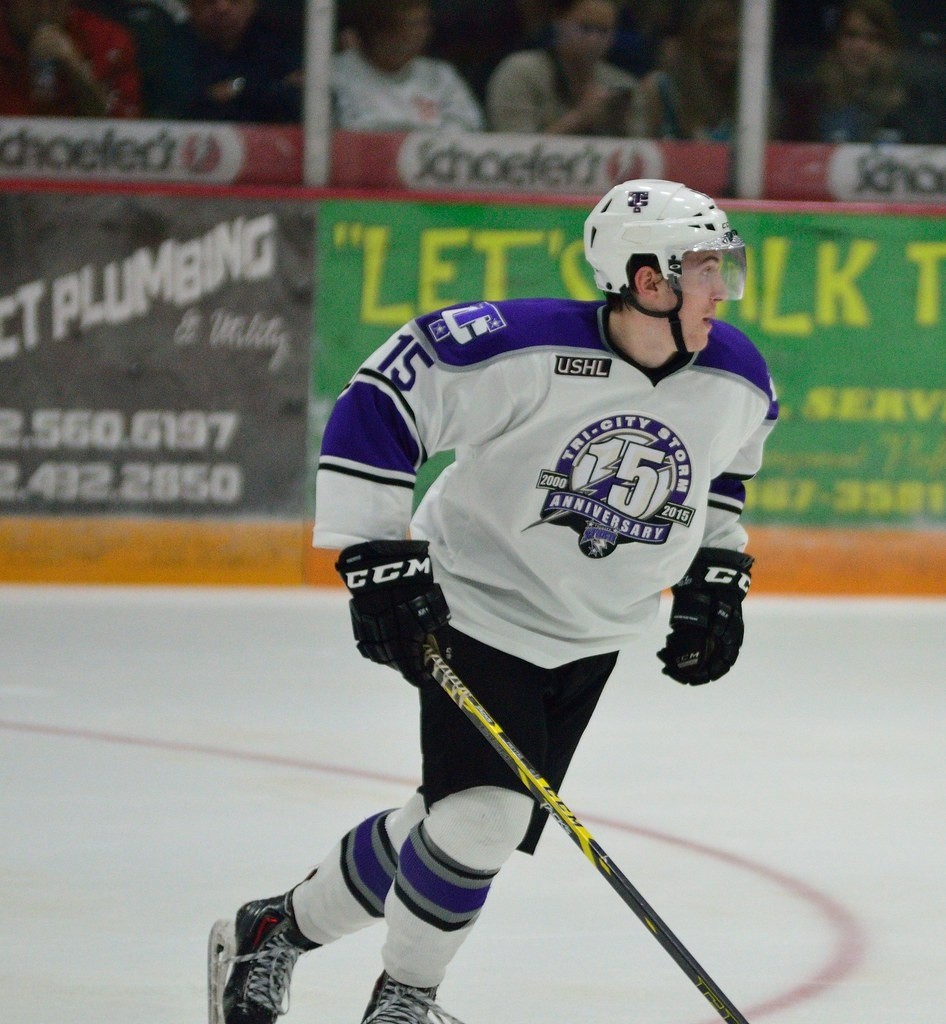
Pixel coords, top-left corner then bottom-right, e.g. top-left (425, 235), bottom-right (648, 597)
top-left (605, 84), bottom-right (633, 120)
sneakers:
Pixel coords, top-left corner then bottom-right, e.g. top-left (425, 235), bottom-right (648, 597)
top-left (207, 869), bottom-right (322, 1024)
top-left (360, 972), bottom-right (465, 1024)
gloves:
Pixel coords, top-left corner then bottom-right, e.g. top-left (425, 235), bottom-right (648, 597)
top-left (657, 549), bottom-right (755, 686)
top-left (334, 539), bottom-right (453, 688)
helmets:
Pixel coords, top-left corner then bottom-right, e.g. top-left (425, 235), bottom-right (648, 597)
top-left (583, 179), bottom-right (745, 295)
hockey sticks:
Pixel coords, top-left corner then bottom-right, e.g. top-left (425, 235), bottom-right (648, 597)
top-left (424, 645), bottom-right (752, 1024)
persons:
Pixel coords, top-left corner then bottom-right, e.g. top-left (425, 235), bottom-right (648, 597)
top-left (0, 0), bottom-right (946, 145)
top-left (210, 179), bottom-right (779, 1024)
